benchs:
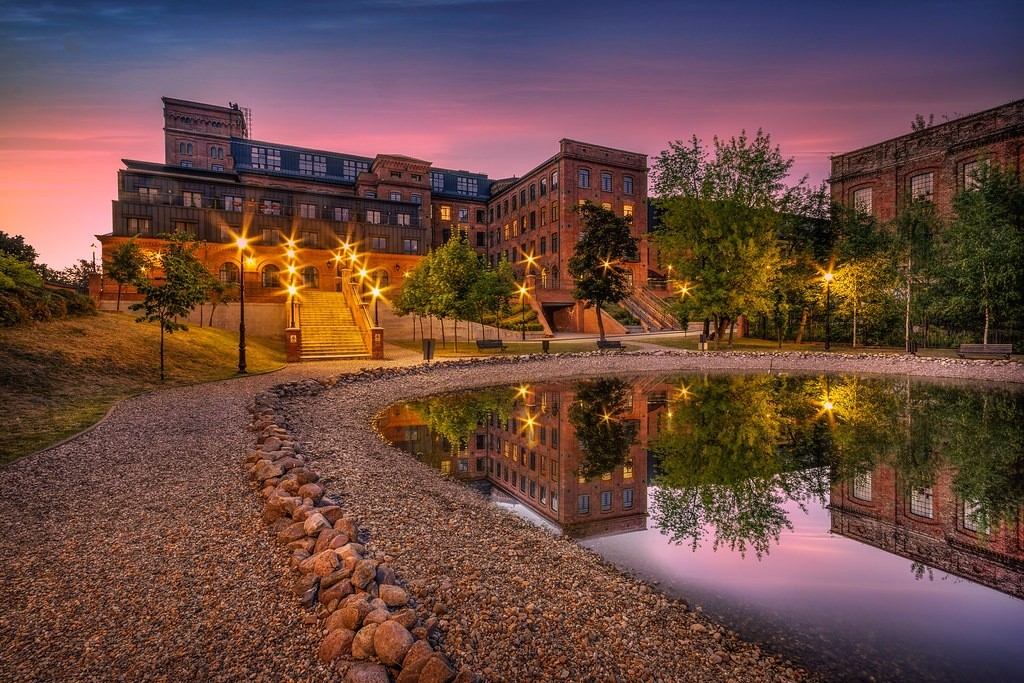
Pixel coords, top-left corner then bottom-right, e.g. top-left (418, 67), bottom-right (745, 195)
top-left (957, 343), bottom-right (1012, 362)
top-left (597, 340), bottom-right (627, 353)
top-left (476, 339), bottom-right (508, 354)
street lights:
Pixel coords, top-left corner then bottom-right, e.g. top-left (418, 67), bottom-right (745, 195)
top-left (824, 273), bottom-right (834, 350)
top-left (825, 374), bottom-right (833, 411)
top-left (518, 286), bottom-right (528, 340)
top-left (235, 236), bottom-right (249, 375)
